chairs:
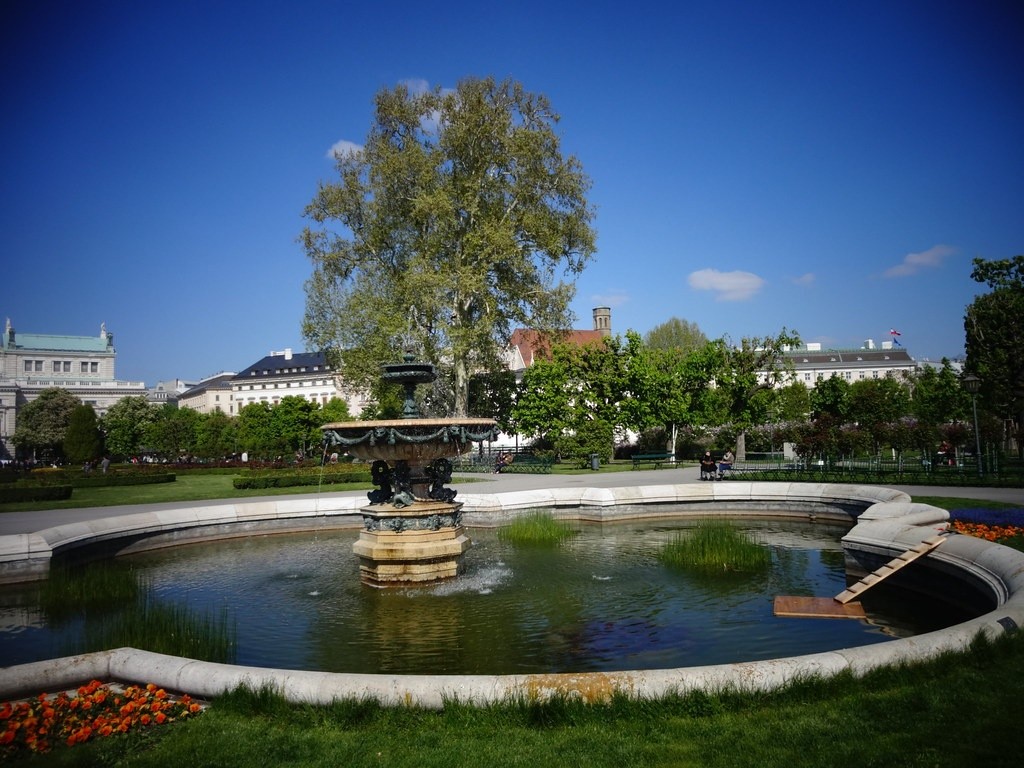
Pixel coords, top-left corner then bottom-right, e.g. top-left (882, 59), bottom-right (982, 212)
top-left (495, 452), bottom-right (553, 474)
top-left (701, 453), bottom-right (1024, 487)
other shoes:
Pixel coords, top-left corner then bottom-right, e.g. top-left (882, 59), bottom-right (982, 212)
top-left (491, 472), bottom-right (495, 474)
top-left (719, 478), bottom-right (724, 481)
top-left (718, 472), bottom-right (724, 475)
top-left (710, 476), bottom-right (714, 481)
top-left (704, 476), bottom-right (708, 480)
top-left (496, 471), bottom-right (499, 474)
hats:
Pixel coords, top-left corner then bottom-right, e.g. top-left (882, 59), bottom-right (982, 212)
top-left (706, 452), bottom-right (710, 456)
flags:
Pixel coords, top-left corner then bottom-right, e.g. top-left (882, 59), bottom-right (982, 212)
top-left (894, 338), bottom-right (902, 346)
top-left (891, 328), bottom-right (901, 336)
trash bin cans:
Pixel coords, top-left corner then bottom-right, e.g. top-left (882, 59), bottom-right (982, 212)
top-left (591, 453), bottom-right (599, 470)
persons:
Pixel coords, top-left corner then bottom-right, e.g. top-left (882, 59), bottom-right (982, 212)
top-left (321, 451), bottom-right (338, 465)
top-left (130, 456), bottom-right (137, 464)
top-left (699, 449), bottom-right (718, 481)
top-left (718, 449), bottom-right (734, 481)
top-left (82, 455), bottom-right (111, 472)
top-left (937, 440), bottom-right (951, 465)
top-left (493, 452), bottom-right (512, 474)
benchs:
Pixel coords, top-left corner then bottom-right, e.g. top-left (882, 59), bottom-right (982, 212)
top-left (631, 454), bottom-right (683, 471)
